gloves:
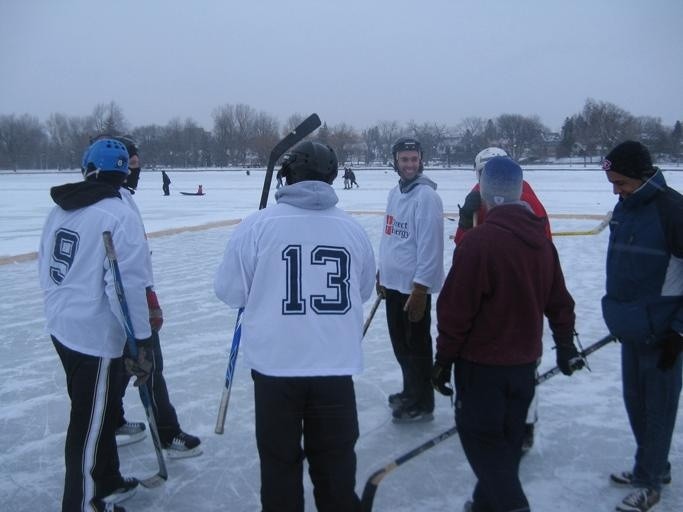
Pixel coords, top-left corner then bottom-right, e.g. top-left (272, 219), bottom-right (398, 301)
top-left (552, 331), bottom-right (585, 376)
top-left (146, 291), bottom-right (163, 332)
top-left (649, 327), bottom-right (683, 370)
top-left (375, 270), bottom-right (388, 299)
top-left (122, 335), bottom-right (155, 386)
top-left (403, 283), bottom-right (427, 323)
top-left (429, 362), bottom-right (453, 396)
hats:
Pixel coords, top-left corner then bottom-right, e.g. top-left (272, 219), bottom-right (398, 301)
top-left (601, 140), bottom-right (655, 179)
top-left (479, 156), bottom-right (523, 208)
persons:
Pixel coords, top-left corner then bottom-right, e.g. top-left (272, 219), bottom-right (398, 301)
top-left (342, 169), bottom-right (351, 189)
top-left (427, 154), bottom-right (585, 512)
top-left (160, 170), bottom-right (171, 196)
top-left (196, 183), bottom-right (202, 195)
top-left (212, 140), bottom-right (379, 512)
top-left (451, 146), bottom-right (555, 247)
top-left (343, 167), bottom-right (348, 176)
top-left (348, 168), bottom-right (359, 189)
top-left (376, 137), bottom-right (446, 423)
top-left (37, 137), bottom-right (155, 511)
top-left (599, 139), bottom-right (683, 512)
top-left (103, 135), bottom-right (207, 459)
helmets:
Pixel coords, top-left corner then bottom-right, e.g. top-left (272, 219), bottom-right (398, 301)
top-left (392, 136), bottom-right (423, 174)
top-left (279, 140), bottom-right (338, 185)
top-left (79, 139), bottom-right (129, 181)
top-left (473, 147), bottom-right (507, 182)
top-left (111, 136), bottom-right (138, 157)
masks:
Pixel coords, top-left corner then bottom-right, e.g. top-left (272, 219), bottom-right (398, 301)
top-left (126, 167), bottom-right (139, 186)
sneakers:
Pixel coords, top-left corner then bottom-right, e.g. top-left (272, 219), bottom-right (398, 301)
top-left (392, 400), bottom-right (433, 420)
top-left (608, 461), bottom-right (671, 485)
top-left (520, 423), bottom-right (533, 452)
top-left (615, 485), bottom-right (659, 512)
top-left (98, 502), bottom-right (125, 512)
top-left (108, 476), bottom-right (139, 494)
top-left (114, 422), bottom-right (145, 435)
top-left (388, 392), bottom-right (410, 406)
top-left (169, 431), bottom-right (200, 451)
